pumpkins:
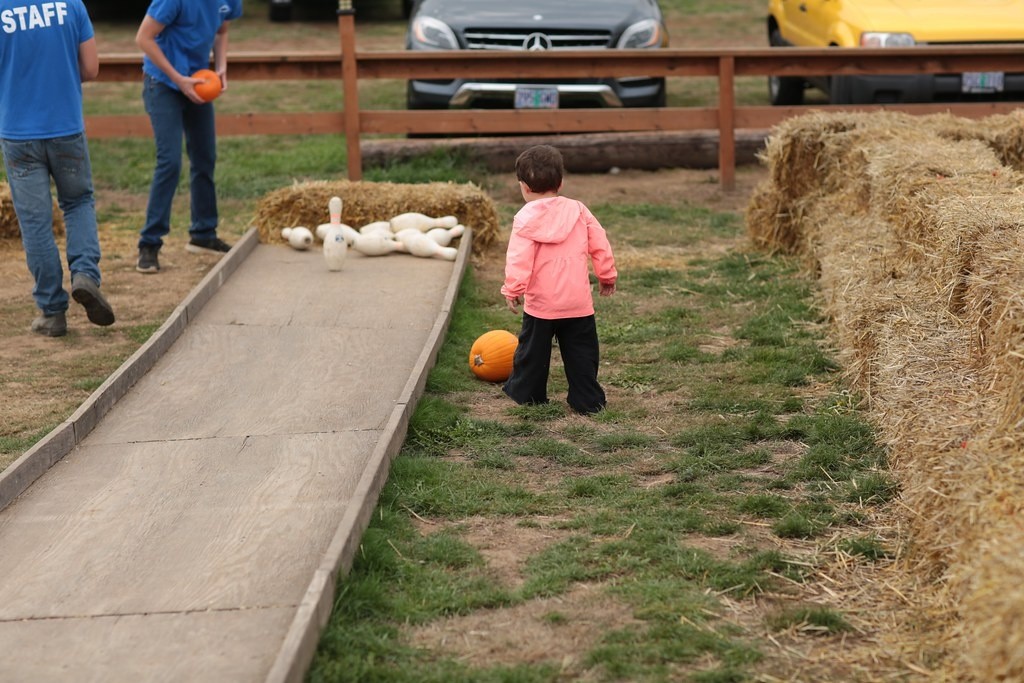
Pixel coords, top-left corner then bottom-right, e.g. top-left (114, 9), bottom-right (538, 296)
top-left (190, 69), bottom-right (222, 100)
top-left (469, 330), bottom-right (518, 381)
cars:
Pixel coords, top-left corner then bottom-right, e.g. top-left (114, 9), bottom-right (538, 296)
top-left (766, 3), bottom-right (1024, 103)
top-left (407, 0), bottom-right (670, 140)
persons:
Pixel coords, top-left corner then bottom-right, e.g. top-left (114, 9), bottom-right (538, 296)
top-left (0, 0), bottom-right (116, 337)
top-left (500, 145), bottom-right (618, 415)
top-left (135, 0), bottom-right (242, 272)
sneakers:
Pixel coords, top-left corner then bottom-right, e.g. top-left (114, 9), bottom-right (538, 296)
top-left (185, 235), bottom-right (232, 255)
top-left (31, 312), bottom-right (67, 336)
top-left (71, 276), bottom-right (114, 326)
top-left (136, 242), bottom-right (161, 273)
